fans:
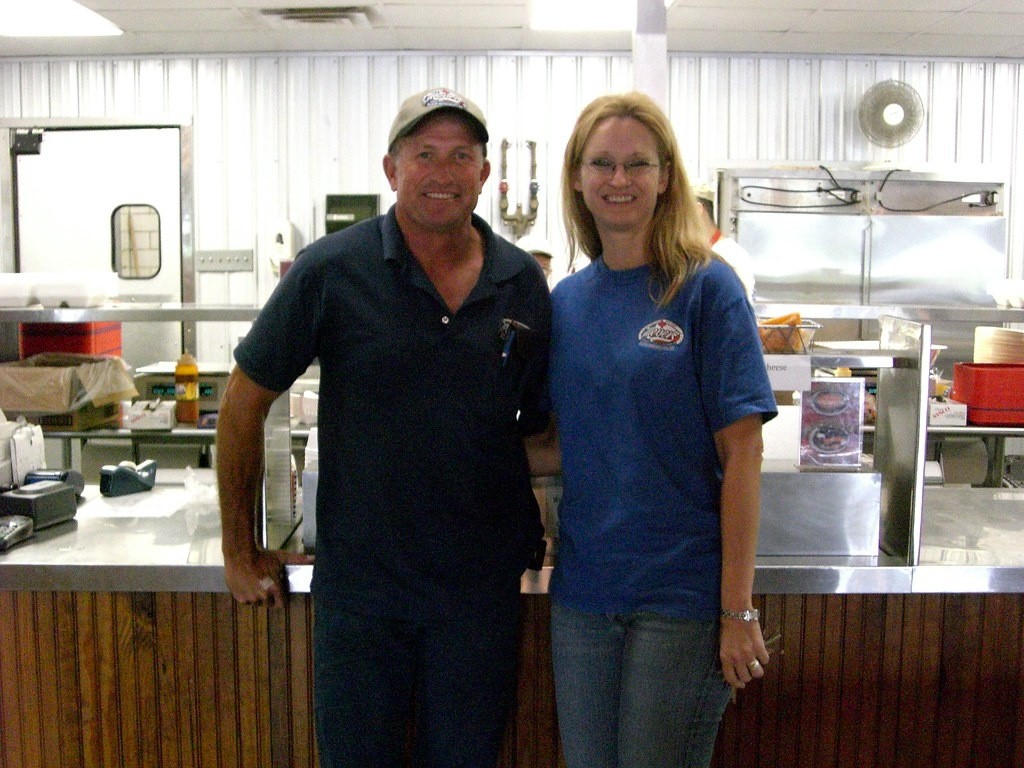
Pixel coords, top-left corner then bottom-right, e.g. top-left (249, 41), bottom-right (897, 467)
top-left (857, 79), bottom-right (924, 149)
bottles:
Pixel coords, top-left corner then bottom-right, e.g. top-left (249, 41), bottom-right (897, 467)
top-left (175, 348), bottom-right (200, 424)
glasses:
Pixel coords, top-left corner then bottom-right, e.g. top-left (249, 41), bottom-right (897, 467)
top-left (541, 266), bottom-right (551, 279)
top-left (575, 156), bottom-right (663, 175)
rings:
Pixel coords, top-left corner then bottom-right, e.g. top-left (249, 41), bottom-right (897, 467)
top-left (748, 659), bottom-right (760, 670)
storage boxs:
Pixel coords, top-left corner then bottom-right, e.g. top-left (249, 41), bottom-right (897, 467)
top-left (952, 362), bottom-right (1024, 424)
top-left (1, 352), bottom-right (138, 435)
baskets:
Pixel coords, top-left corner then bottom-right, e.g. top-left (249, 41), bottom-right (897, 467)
top-left (756, 318), bottom-right (823, 355)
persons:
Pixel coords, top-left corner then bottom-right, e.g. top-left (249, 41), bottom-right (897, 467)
top-left (216, 88), bottom-right (552, 768)
top-left (523, 91), bottom-right (777, 768)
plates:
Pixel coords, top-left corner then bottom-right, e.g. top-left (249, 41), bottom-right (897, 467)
top-left (973, 325), bottom-right (1024, 363)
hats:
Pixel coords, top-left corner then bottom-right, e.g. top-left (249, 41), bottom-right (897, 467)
top-left (388, 88), bottom-right (489, 154)
top-left (515, 235), bottom-right (554, 258)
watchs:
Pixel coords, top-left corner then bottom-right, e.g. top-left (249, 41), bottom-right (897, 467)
top-left (721, 609), bottom-right (762, 621)
